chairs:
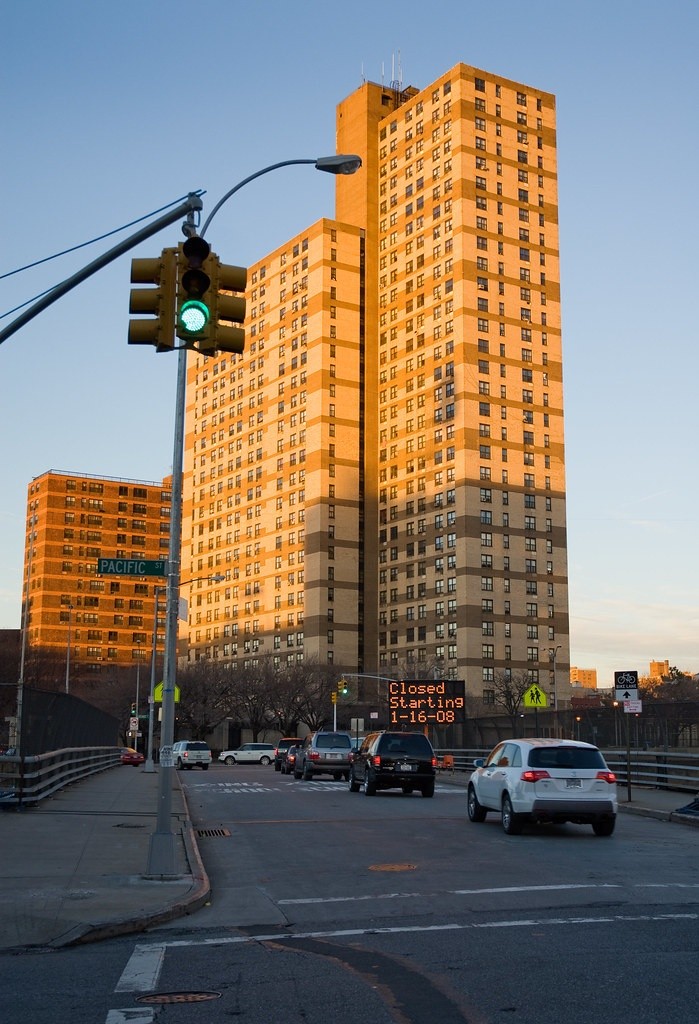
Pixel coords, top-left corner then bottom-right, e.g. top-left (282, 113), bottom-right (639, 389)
top-left (555, 751), bottom-right (572, 767)
top-left (381, 743), bottom-right (388, 751)
top-left (529, 756), bottom-right (542, 766)
top-left (390, 744), bottom-right (401, 751)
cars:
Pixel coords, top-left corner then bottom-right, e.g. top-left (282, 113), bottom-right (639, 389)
top-left (173, 740), bottom-right (212, 770)
top-left (351, 736), bottom-right (365, 752)
top-left (218, 743), bottom-right (277, 765)
top-left (281, 745), bottom-right (302, 774)
top-left (274, 738), bottom-right (305, 771)
top-left (467, 738), bottom-right (618, 837)
top-left (121, 747), bottom-right (145, 767)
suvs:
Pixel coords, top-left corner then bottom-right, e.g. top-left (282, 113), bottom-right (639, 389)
top-left (349, 730), bottom-right (437, 797)
top-left (294, 731), bottom-right (356, 782)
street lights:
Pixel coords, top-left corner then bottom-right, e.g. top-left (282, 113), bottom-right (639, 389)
top-left (541, 646), bottom-right (562, 710)
top-left (139, 154), bottom-right (360, 880)
top-left (66, 605), bottom-right (73, 694)
top-left (143, 576), bottom-right (225, 773)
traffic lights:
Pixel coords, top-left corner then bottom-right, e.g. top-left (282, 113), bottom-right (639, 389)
top-left (128, 247), bottom-right (177, 352)
top-left (198, 252), bottom-right (247, 357)
top-left (176, 237), bottom-right (220, 342)
top-left (332, 693), bottom-right (336, 703)
top-left (338, 680), bottom-right (343, 693)
top-left (131, 703), bottom-right (136, 714)
top-left (342, 681), bottom-right (348, 693)
